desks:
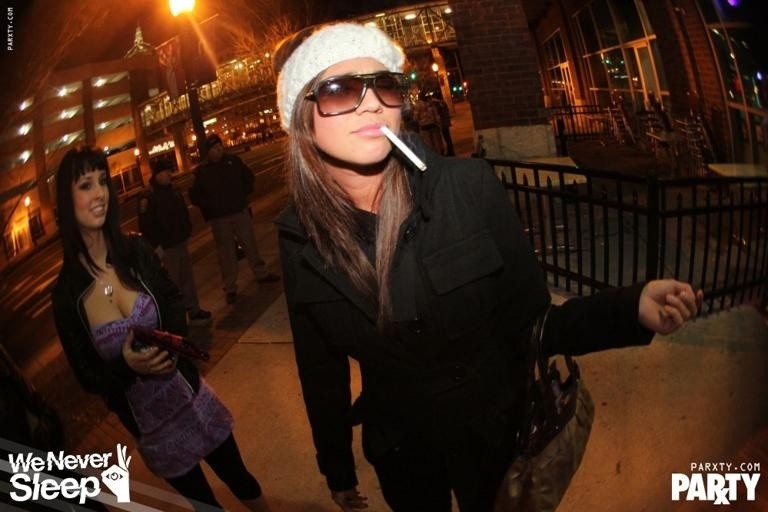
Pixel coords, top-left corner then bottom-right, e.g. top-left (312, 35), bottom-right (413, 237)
top-left (491, 157), bottom-right (588, 256)
top-left (645, 130), bottom-right (703, 143)
top-left (708, 162), bottom-right (768, 250)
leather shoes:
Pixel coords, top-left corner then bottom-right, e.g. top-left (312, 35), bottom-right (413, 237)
top-left (226, 290), bottom-right (237, 304)
top-left (256, 274), bottom-right (279, 284)
top-left (189, 310), bottom-right (212, 321)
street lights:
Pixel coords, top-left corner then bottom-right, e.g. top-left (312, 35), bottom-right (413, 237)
top-left (133, 149), bottom-right (145, 189)
top-left (191, 134), bottom-right (200, 159)
top-left (431, 62), bottom-right (442, 101)
top-left (170, 0), bottom-right (209, 157)
top-left (24, 196), bottom-right (38, 248)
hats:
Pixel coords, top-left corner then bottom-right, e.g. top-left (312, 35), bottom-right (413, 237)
top-left (202, 133), bottom-right (223, 156)
top-left (277, 22), bottom-right (409, 135)
top-left (153, 158), bottom-right (174, 175)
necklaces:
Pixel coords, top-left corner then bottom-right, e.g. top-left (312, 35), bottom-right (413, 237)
top-left (93, 268), bottom-right (113, 297)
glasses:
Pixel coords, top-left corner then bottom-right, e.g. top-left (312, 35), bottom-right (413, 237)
top-left (306, 71), bottom-right (407, 118)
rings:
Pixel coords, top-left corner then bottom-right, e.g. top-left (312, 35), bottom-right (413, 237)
top-left (144, 360), bottom-right (151, 370)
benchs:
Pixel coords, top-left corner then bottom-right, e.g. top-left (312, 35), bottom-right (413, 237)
top-left (473, 124), bottom-right (557, 161)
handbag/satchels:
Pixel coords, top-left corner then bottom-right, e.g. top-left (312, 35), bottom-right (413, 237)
top-left (494, 361), bottom-right (595, 512)
top-left (127, 325), bottom-right (197, 371)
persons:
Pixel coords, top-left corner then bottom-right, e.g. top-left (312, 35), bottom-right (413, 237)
top-left (267, 17), bottom-right (704, 510)
top-left (136, 158), bottom-right (213, 320)
top-left (0, 345), bottom-right (74, 455)
top-left (47, 144), bottom-right (273, 511)
top-left (430, 90), bottom-right (457, 157)
top-left (413, 91), bottom-right (446, 157)
top-left (379, 127), bottom-right (426, 172)
top-left (186, 133), bottom-right (279, 308)
